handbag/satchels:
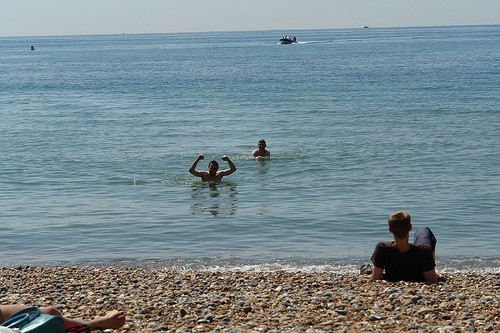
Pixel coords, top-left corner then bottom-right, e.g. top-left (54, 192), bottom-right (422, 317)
top-left (0, 307), bottom-right (65, 333)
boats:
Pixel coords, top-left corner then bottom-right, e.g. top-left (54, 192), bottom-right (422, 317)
top-left (279, 35), bottom-right (298, 45)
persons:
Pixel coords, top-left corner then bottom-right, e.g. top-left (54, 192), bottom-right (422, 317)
top-left (0, 305), bottom-right (126, 333)
top-left (283, 35), bottom-right (296, 41)
top-left (370, 211), bottom-right (435, 282)
top-left (252, 139), bottom-right (270, 161)
top-left (189, 154), bottom-right (236, 182)
